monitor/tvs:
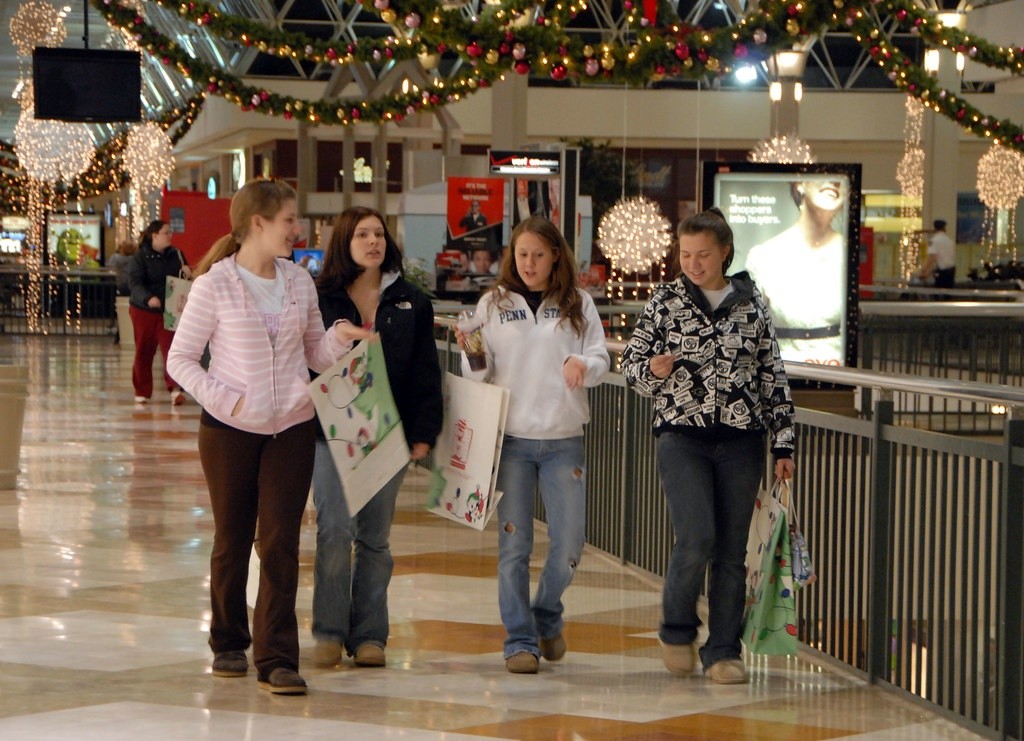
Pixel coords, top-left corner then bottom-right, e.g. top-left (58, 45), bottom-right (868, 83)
top-left (32, 47), bottom-right (142, 123)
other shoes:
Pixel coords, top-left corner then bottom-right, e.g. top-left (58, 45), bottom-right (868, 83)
top-left (355, 642), bottom-right (385, 665)
top-left (211, 653), bottom-right (249, 676)
top-left (170, 391), bottom-right (186, 406)
top-left (134, 396), bottom-right (150, 403)
top-left (312, 640), bottom-right (343, 665)
top-left (256, 666), bottom-right (307, 693)
top-left (539, 634), bottom-right (567, 661)
top-left (706, 659), bottom-right (748, 684)
top-left (505, 650), bottom-right (538, 673)
top-left (658, 638), bottom-right (695, 675)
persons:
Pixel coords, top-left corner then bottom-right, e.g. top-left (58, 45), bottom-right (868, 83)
top-left (918, 219), bottom-right (957, 287)
top-left (454, 217), bottom-right (610, 673)
top-left (460, 200), bottom-right (486, 233)
top-left (744, 176), bottom-right (846, 365)
top-left (126, 218), bottom-right (192, 407)
top-left (438, 245), bottom-right (508, 292)
top-left (617, 206), bottom-right (796, 685)
top-left (311, 205), bottom-right (444, 667)
top-left (167, 178), bottom-right (374, 696)
top-left (107, 241), bottom-right (135, 345)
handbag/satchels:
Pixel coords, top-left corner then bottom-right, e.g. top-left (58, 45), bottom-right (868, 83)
top-left (305, 332), bottom-right (411, 517)
top-left (163, 269), bottom-right (193, 331)
top-left (425, 369), bottom-right (511, 532)
top-left (739, 476), bottom-right (798, 656)
top-left (782, 478), bottom-right (818, 591)
